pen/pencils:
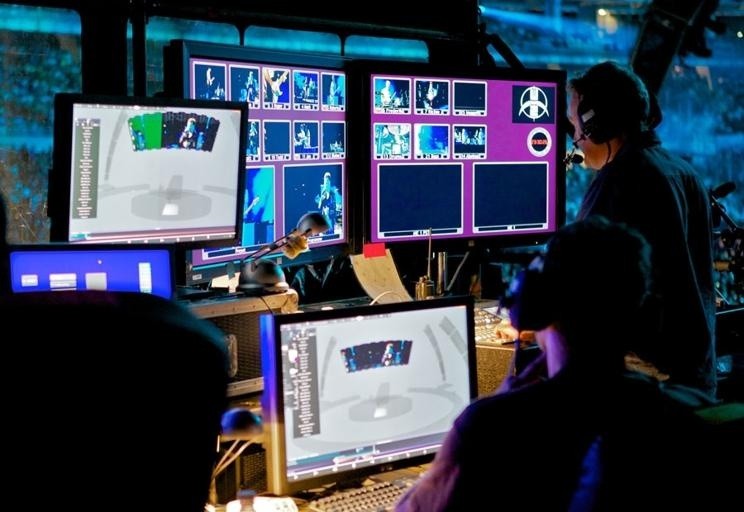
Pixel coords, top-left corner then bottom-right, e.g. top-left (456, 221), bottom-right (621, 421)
top-left (438, 252), bottom-right (447, 297)
top-left (446, 251), bottom-right (469, 291)
top-left (427, 236), bottom-right (431, 279)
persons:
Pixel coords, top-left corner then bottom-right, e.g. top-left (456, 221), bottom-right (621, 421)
top-left (289, 212), bottom-right (373, 302)
top-left (567, 60), bottom-right (716, 400)
top-left (392, 217), bottom-right (744, 512)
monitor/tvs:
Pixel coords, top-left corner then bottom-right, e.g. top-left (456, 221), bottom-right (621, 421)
top-left (345, 59), bottom-right (566, 256)
top-left (164, 40), bottom-right (360, 285)
top-left (50, 93), bottom-right (245, 248)
top-left (260, 294), bottom-right (479, 495)
top-left (8, 244), bottom-right (172, 294)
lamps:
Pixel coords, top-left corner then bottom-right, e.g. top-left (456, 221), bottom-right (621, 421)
top-left (236, 212), bottom-right (328, 293)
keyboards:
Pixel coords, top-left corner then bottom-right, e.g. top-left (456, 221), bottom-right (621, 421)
top-left (308, 469), bottom-right (432, 511)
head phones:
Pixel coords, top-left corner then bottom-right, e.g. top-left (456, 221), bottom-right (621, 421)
top-left (565, 60), bottom-right (663, 167)
